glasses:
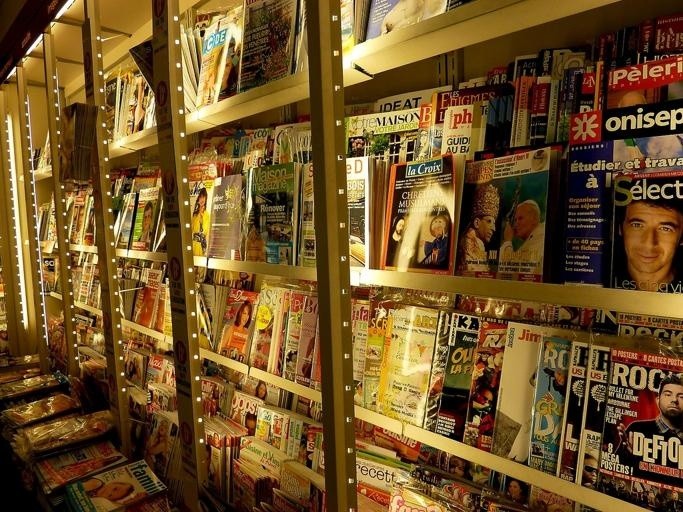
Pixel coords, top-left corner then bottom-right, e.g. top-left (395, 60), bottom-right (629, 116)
top-left (483, 395), bottom-right (492, 403)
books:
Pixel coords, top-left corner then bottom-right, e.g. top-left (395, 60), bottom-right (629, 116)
top-left (2, 1), bottom-right (681, 512)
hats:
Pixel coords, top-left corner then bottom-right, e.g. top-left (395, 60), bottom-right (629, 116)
top-left (471, 184), bottom-right (500, 219)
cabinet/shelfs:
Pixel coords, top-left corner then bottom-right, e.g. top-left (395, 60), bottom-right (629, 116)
top-left (0, 0), bottom-right (683, 512)
